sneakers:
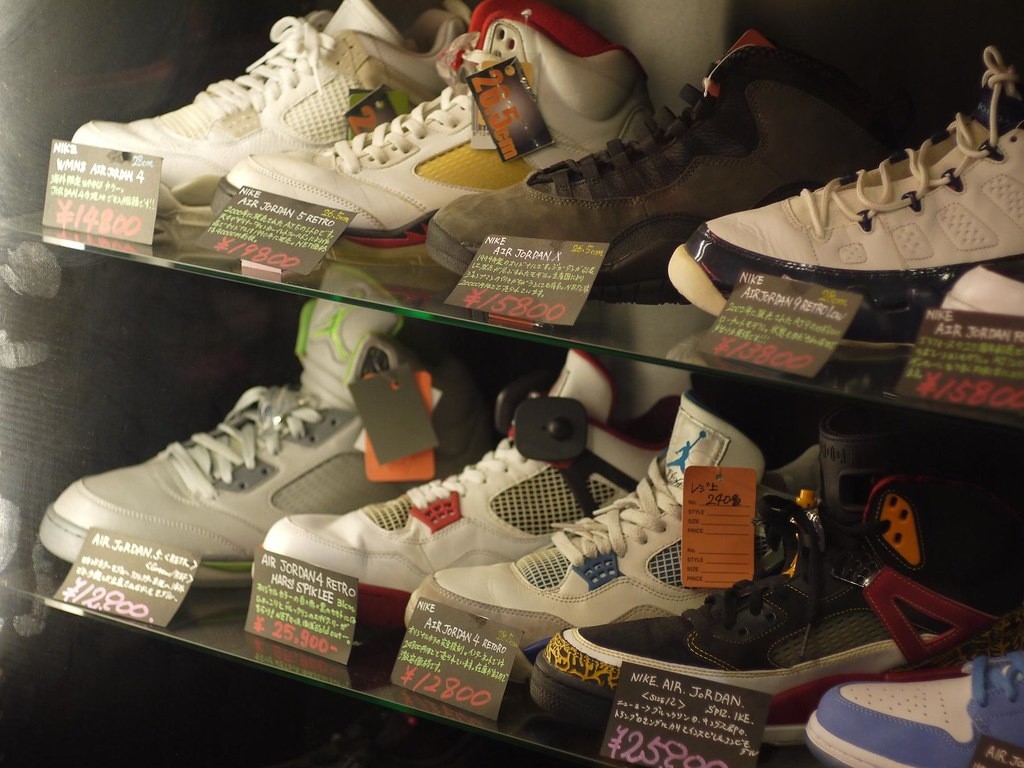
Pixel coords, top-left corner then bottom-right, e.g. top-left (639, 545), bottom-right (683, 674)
top-left (39, 0), bottom-right (1024, 768)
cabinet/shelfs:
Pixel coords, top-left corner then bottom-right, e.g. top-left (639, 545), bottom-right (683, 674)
top-left (0, 210), bottom-right (1024, 768)
